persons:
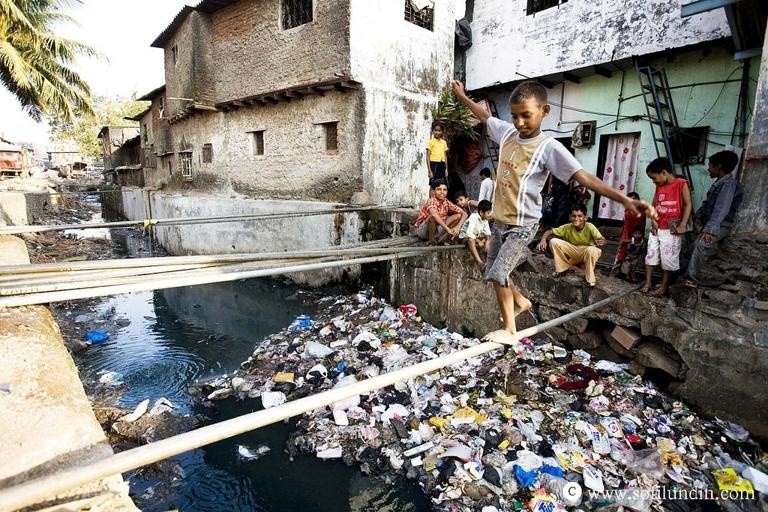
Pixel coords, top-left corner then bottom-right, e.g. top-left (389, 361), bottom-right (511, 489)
top-left (639, 156), bottom-right (692, 297)
top-left (680, 150), bottom-right (744, 308)
top-left (453, 191), bottom-right (478, 215)
top-left (610, 191), bottom-right (652, 276)
top-left (414, 179), bottom-right (468, 245)
top-left (478, 167), bottom-right (494, 203)
top-left (451, 79), bottom-right (660, 347)
top-left (425, 121), bottom-right (449, 198)
top-left (536, 202), bottom-right (608, 287)
top-left (621, 227), bottom-right (645, 281)
top-left (458, 200), bottom-right (492, 270)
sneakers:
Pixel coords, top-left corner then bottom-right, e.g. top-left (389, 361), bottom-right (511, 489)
top-left (654, 288), bottom-right (667, 294)
top-left (426, 240), bottom-right (450, 246)
top-left (552, 268), bottom-right (575, 278)
top-left (641, 284), bottom-right (651, 292)
